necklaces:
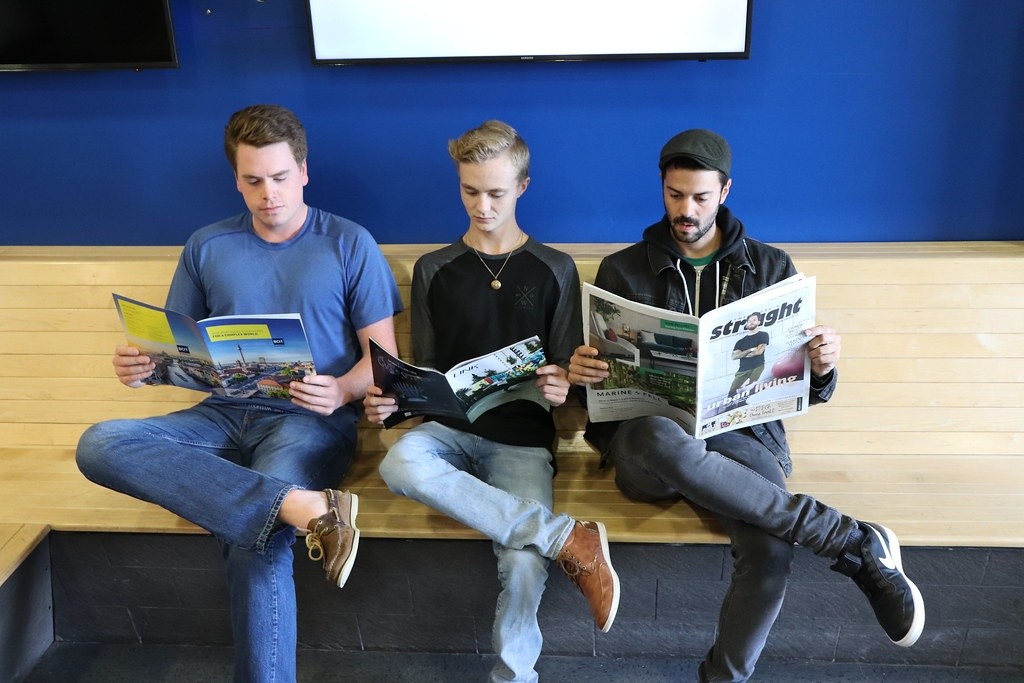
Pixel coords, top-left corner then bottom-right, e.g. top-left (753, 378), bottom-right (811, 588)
top-left (467, 225), bottom-right (526, 292)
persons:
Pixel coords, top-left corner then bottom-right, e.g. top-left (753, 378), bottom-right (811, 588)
top-left (74, 103), bottom-right (405, 680)
top-left (568, 131), bottom-right (927, 680)
top-left (726, 310), bottom-right (769, 410)
top-left (363, 117), bottom-right (619, 680)
top-left (483, 371), bottom-right (512, 393)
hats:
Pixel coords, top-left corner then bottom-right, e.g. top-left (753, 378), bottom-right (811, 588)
top-left (659, 129), bottom-right (731, 178)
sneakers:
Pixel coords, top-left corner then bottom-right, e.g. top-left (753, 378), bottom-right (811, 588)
top-left (829, 520), bottom-right (925, 648)
top-left (293, 488), bottom-right (360, 588)
top-left (556, 520), bottom-right (620, 633)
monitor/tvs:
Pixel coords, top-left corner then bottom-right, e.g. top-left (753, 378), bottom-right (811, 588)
top-left (0, 0), bottom-right (181, 73)
top-left (305, 1), bottom-right (755, 66)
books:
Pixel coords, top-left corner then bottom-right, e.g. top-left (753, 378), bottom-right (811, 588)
top-left (366, 332), bottom-right (553, 424)
top-left (109, 290), bottom-right (321, 402)
top-left (581, 272), bottom-right (815, 439)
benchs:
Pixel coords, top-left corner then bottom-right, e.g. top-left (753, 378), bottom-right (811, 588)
top-left (0, 241), bottom-right (1024, 594)
